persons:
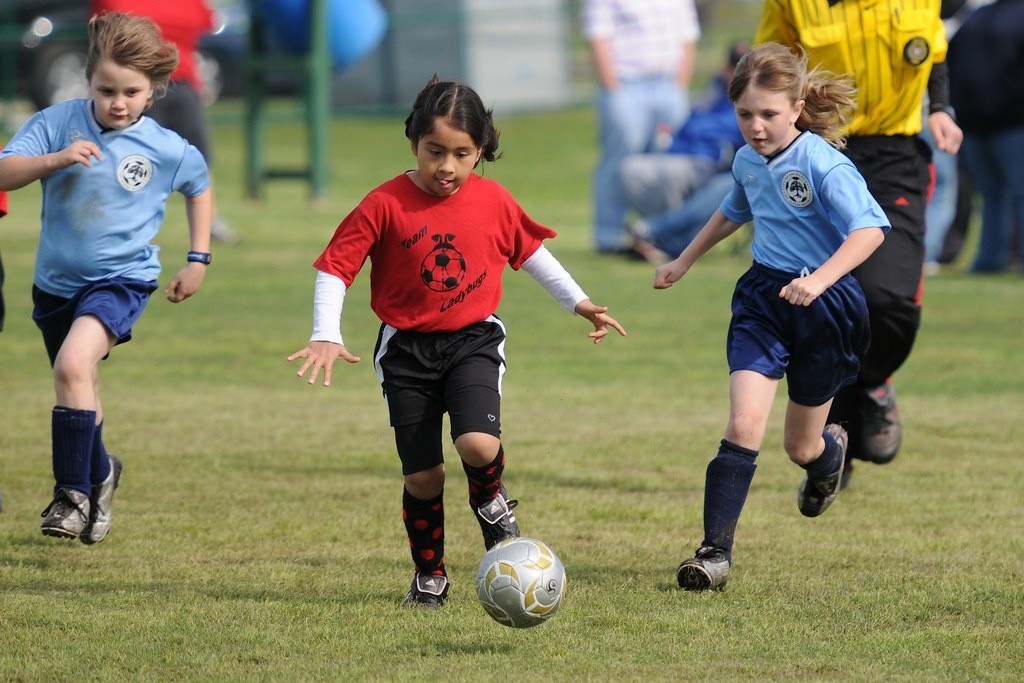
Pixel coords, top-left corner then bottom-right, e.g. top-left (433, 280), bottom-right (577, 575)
top-left (584, 0), bottom-right (755, 266)
top-left (92, 0), bottom-right (243, 246)
top-left (654, 41), bottom-right (892, 593)
top-left (0, 13), bottom-right (211, 544)
top-left (286, 82), bottom-right (626, 610)
top-left (751, 3), bottom-right (962, 463)
top-left (920, 1), bottom-right (1023, 275)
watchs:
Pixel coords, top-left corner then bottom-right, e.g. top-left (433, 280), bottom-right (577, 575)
top-left (187, 251), bottom-right (211, 265)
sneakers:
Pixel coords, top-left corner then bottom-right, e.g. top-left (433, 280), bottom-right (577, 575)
top-left (858, 377), bottom-right (902, 464)
top-left (470, 484), bottom-right (520, 552)
top-left (79, 455), bottom-right (121, 544)
top-left (798, 423), bottom-right (849, 518)
top-left (677, 541), bottom-right (730, 594)
top-left (402, 572), bottom-right (450, 613)
top-left (40, 485), bottom-right (91, 540)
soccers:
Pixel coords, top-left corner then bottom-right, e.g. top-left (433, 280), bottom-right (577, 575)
top-left (475, 534), bottom-right (568, 629)
